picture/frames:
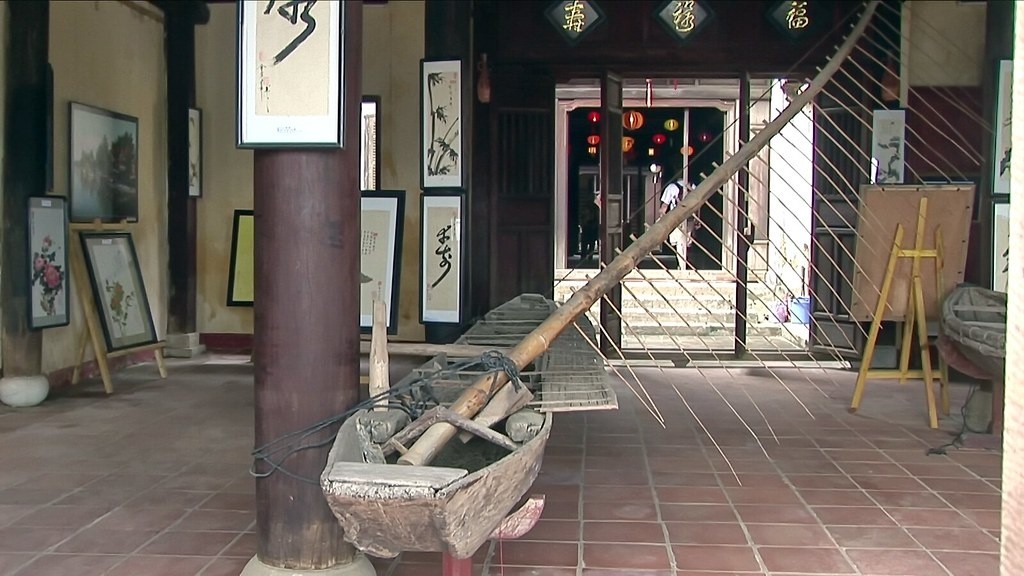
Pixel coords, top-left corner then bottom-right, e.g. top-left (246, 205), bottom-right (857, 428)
top-left (24, 193), bottom-right (69, 332)
top-left (868, 107), bottom-right (906, 185)
top-left (68, 100), bottom-right (139, 224)
top-left (236, 0), bottom-right (345, 152)
top-left (418, 193), bottom-right (464, 325)
top-left (986, 56), bottom-right (1012, 197)
top-left (990, 199), bottom-right (1007, 295)
top-left (419, 57), bottom-right (467, 190)
top-left (356, 190), bottom-right (407, 335)
top-left (184, 104), bottom-right (204, 198)
top-left (226, 208), bottom-right (256, 308)
top-left (78, 231), bottom-right (158, 354)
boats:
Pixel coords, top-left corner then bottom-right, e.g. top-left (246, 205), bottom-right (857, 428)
top-left (318, 289), bottom-right (560, 560)
top-left (939, 279), bottom-right (1007, 384)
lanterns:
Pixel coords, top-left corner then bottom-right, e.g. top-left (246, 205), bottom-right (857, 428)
top-left (664, 119), bottom-right (679, 130)
top-left (588, 111), bottom-right (601, 122)
top-left (652, 134), bottom-right (666, 144)
top-left (650, 163), bottom-right (661, 184)
top-left (622, 111), bottom-right (643, 131)
top-left (588, 147), bottom-right (596, 154)
top-left (648, 148), bottom-right (657, 156)
top-left (622, 137), bottom-right (634, 152)
top-left (587, 135), bottom-right (600, 145)
top-left (681, 145), bottom-right (694, 155)
top-left (698, 132), bottom-right (712, 141)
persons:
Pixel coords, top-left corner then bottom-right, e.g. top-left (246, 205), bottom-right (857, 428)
top-left (657, 166), bottom-right (701, 270)
top-left (579, 191), bottom-right (600, 261)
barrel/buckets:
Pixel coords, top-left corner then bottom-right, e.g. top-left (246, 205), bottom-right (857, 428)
top-left (791, 298), bottom-right (810, 323)
top-left (769, 302), bottom-right (788, 323)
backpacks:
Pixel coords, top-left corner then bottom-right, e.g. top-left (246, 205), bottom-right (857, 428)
top-left (670, 181), bottom-right (696, 223)
